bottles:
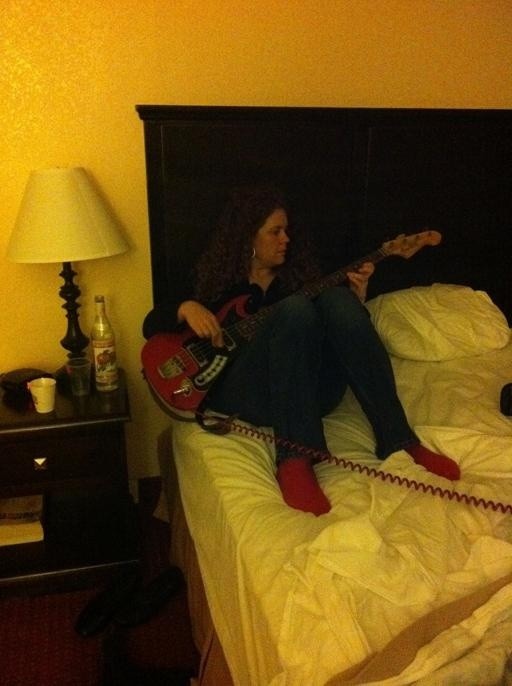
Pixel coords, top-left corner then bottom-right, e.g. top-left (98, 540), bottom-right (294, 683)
top-left (90, 294), bottom-right (120, 392)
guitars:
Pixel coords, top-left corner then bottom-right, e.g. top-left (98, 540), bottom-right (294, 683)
top-left (140, 229), bottom-right (444, 424)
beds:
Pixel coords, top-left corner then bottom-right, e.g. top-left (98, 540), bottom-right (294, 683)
top-left (134, 103), bottom-right (512, 686)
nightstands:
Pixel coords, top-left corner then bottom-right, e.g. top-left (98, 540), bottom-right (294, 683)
top-left (0, 368), bottom-right (141, 597)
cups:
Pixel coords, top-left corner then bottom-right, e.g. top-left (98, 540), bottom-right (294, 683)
top-left (28, 377), bottom-right (58, 415)
top-left (64, 357), bottom-right (93, 398)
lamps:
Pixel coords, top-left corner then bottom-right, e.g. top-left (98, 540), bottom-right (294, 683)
top-left (4, 164), bottom-right (130, 390)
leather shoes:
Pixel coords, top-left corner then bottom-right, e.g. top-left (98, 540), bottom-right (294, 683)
top-left (116, 564), bottom-right (185, 628)
top-left (74, 569), bottom-right (143, 639)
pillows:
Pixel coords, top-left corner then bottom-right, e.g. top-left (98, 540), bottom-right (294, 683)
top-left (363, 281), bottom-right (509, 361)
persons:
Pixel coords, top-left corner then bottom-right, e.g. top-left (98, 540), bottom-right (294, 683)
top-left (143, 197), bottom-right (461, 516)
